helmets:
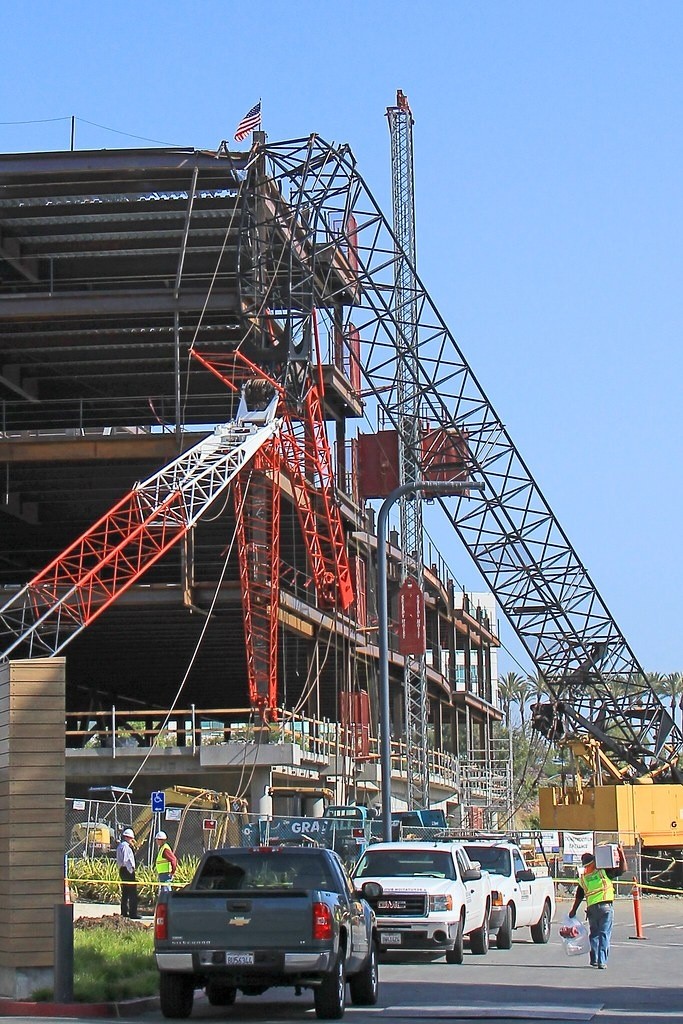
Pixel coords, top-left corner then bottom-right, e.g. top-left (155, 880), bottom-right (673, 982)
top-left (154, 831), bottom-right (167, 840)
top-left (122, 828), bottom-right (135, 839)
top-left (581, 853), bottom-right (595, 867)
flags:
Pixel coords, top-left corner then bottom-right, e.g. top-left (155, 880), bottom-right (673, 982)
top-left (233, 101), bottom-right (262, 142)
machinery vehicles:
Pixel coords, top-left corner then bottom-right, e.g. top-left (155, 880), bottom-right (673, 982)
top-left (237, 134), bottom-right (682, 891)
top-left (70, 786), bottom-right (248, 861)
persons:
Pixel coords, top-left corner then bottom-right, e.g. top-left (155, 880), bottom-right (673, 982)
top-left (569, 845), bottom-right (628, 970)
top-left (154, 831), bottom-right (177, 896)
top-left (298, 864), bottom-right (323, 890)
top-left (116, 828), bottom-right (142, 919)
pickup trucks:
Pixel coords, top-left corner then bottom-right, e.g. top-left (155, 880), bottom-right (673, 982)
top-left (461, 842), bottom-right (556, 949)
top-left (349, 842), bottom-right (492, 963)
top-left (154, 848), bottom-right (384, 1019)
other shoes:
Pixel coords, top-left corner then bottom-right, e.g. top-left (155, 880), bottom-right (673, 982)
top-left (591, 963), bottom-right (608, 969)
top-left (123, 915), bottom-right (142, 919)
